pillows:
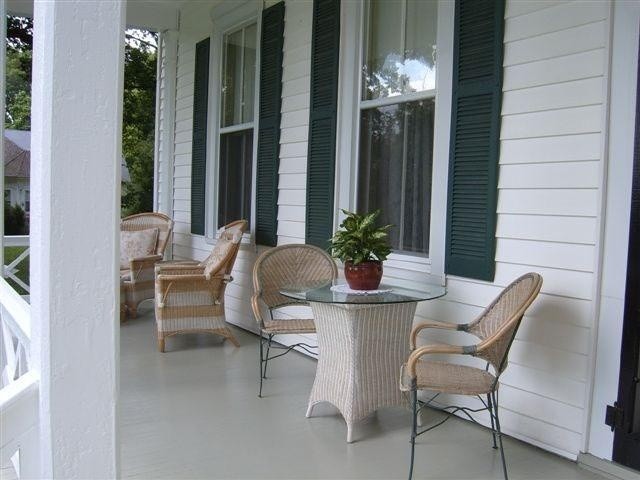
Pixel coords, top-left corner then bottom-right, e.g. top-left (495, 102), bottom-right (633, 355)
top-left (203, 235), bottom-right (233, 276)
top-left (120, 225), bottom-right (160, 271)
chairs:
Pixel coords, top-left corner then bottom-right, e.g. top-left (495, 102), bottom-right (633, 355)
top-left (247, 242), bottom-right (340, 399)
top-left (396, 270), bottom-right (544, 479)
top-left (116, 211), bottom-right (178, 325)
top-left (152, 216), bottom-right (252, 353)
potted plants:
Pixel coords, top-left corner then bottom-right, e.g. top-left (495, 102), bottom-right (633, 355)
top-left (321, 206), bottom-right (398, 292)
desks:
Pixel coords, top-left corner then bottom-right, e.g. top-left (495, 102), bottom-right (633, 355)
top-left (153, 258), bottom-right (202, 267)
top-left (277, 277), bottom-right (452, 446)
